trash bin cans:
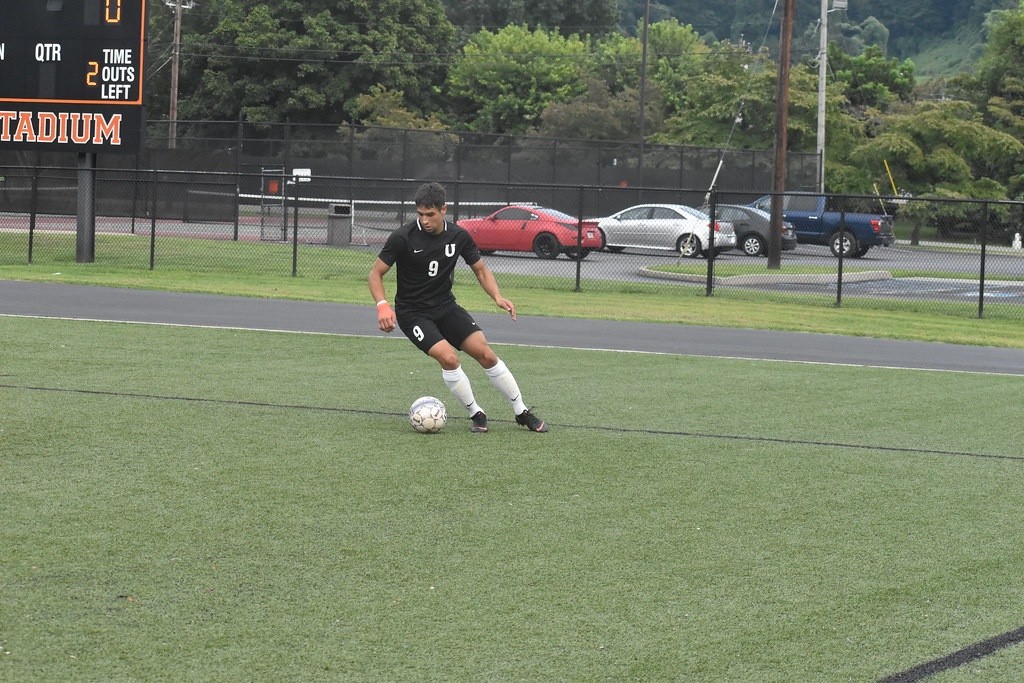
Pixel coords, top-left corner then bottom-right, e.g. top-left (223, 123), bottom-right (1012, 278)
top-left (327, 204), bottom-right (351, 248)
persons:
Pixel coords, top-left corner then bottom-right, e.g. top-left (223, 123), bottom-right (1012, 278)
top-left (366, 183), bottom-right (548, 433)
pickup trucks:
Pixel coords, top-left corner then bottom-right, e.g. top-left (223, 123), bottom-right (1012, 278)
top-left (743, 190), bottom-right (896, 261)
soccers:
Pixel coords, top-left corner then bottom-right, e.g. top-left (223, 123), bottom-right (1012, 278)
top-left (408, 395), bottom-right (447, 434)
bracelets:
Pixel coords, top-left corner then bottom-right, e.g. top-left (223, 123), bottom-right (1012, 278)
top-left (376, 300), bottom-right (388, 306)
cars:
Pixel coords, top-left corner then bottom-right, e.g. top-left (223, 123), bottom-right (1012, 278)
top-left (583, 203), bottom-right (738, 260)
top-left (692, 203), bottom-right (796, 258)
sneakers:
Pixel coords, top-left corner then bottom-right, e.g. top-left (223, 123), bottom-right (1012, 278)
top-left (471, 411), bottom-right (488, 433)
top-left (516, 405), bottom-right (548, 432)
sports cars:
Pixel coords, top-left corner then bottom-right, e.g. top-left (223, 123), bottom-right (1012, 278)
top-left (451, 204), bottom-right (603, 261)
